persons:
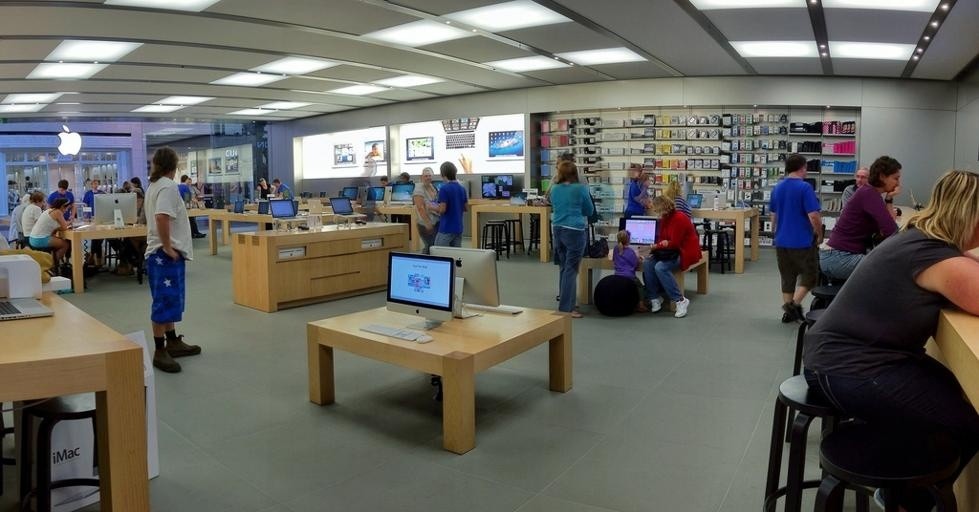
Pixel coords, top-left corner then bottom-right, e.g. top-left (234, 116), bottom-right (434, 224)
top-left (142, 147), bottom-right (202, 374)
top-left (544, 153), bottom-right (591, 205)
top-left (178, 175), bottom-right (205, 238)
top-left (411, 167), bottom-right (439, 255)
top-left (548, 162), bottom-right (595, 319)
top-left (22, 191), bottom-right (47, 244)
top-left (47, 178), bottom-right (76, 264)
top-left (8, 193), bottom-right (29, 249)
top-left (612, 229), bottom-right (649, 314)
top-left (28, 196), bottom-right (77, 276)
top-left (426, 161), bottom-right (468, 247)
top-left (378, 176), bottom-right (389, 186)
top-left (81, 178), bottom-right (106, 268)
top-left (365, 143), bottom-right (382, 160)
top-left (840, 167), bottom-right (872, 212)
top-left (187, 178), bottom-right (200, 208)
top-left (799, 170), bottom-right (979, 510)
top-left (398, 172), bottom-right (411, 184)
top-left (24, 175), bottom-right (33, 192)
top-left (113, 180), bottom-right (132, 193)
top-left (769, 153), bottom-right (823, 325)
top-left (642, 195), bottom-right (702, 319)
top-left (819, 156), bottom-right (903, 280)
top-left (271, 179), bottom-right (295, 200)
top-left (660, 181), bottom-right (692, 218)
top-left (623, 172), bottom-right (651, 232)
top-left (8, 180), bottom-right (20, 214)
top-left (127, 188), bottom-right (146, 224)
top-left (257, 178), bottom-right (276, 200)
top-left (130, 177), bottom-right (145, 194)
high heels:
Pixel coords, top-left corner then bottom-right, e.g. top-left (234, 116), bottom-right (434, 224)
top-left (882, 483), bottom-right (939, 512)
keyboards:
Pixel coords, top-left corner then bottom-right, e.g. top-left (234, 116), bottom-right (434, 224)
top-left (463, 303), bottom-right (523, 315)
top-left (361, 323), bottom-right (423, 342)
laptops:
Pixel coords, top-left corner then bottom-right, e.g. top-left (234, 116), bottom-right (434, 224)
top-left (403, 135), bottom-right (437, 164)
top-left (485, 129), bottom-right (524, 162)
top-left (442, 117), bottom-right (481, 150)
top-left (0, 296), bottom-right (55, 320)
top-left (207, 156), bottom-right (223, 176)
top-left (224, 156), bottom-right (240, 175)
top-left (331, 143), bottom-right (360, 169)
top-left (257, 201), bottom-right (268, 214)
top-left (234, 200), bottom-right (244, 214)
top-left (268, 199), bottom-right (300, 219)
top-left (362, 139), bottom-right (387, 167)
top-left (330, 196), bottom-right (358, 215)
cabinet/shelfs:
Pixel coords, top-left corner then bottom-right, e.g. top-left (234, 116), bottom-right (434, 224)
top-left (537, 104), bottom-right (861, 248)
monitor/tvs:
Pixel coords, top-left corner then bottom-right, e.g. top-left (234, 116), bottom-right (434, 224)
top-left (618, 216), bottom-right (659, 256)
top-left (387, 251), bottom-right (455, 332)
top-left (480, 175), bottom-right (514, 200)
top-left (429, 243), bottom-right (499, 320)
top-left (367, 187), bottom-right (386, 207)
top-left (431, 180), bottom-right (444, 193)
top-left (342, 187), bottom-right (358, 201)
top-left (686, 194), bottom-right (703, 209)
top-left (94, 192), bottom-right (138, 230)
top-left (390, 183), bottom-right (415, 207)
top-left (293, 201), bottom-right (298, 215)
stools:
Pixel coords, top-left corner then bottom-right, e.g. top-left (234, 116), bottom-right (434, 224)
top-left (809, 286), bottom-right (841, 311)
top-left (20, 390), bottom-right (102, 511)
top-left (12, 233), bottom-right (126, 278)
top-left (481, 217), bottom-right (526, 261)
top-left (813, 418), bottom-right (963, 512)
top-left (785, 308), bottom-right (830, 442)
top-left (764, 373), bottom-right (874, 511)
top-left (692, 221), bottom-right (736, 275)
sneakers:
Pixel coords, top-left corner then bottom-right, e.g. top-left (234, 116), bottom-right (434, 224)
top-left (674, 296), bottom-right (689, 318)
top-left (153, 350), bottom-right (181, 372)
top-left (166, 335), bottom-right (200, 357)
top-left (782, 313), bottom-right (792, 322)
top-left (651, 296), bottom-right (664, 312)
top-left (569, 309), bottom-right (583, 318)
top-left (113, 266), bottom-right (134, 275)
top-left (783, 301), bottom-right (805, 324)
top-left (639, 304), bottom-right (649, 312)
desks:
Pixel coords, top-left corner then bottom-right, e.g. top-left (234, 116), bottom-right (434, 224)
top-left (306, 305), bottom-right (575, 455)
top-left (50, 221), bottom-right (148, 295)
top-left (184, 207), bottom-right (230, 247)
top-left (471, 203), bottom-right (552, 264)
top-left (1, 289), bottom-right (151, 511)
top-left (355, 200), bottom-right (424, 251)
top-left (646, 207), bottom-right (759, 274)
top-left (578, 249), bottom-right (709, 312)
top-left (232, 222), bottom-right (409, 313)
top-left (208, 213), bottom-right (368, 255)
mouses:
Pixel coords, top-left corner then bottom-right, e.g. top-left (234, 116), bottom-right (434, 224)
top-left (417, 336), bottom-right (433, 344)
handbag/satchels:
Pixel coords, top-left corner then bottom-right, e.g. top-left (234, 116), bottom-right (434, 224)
top-left (654, 248), bottom-right (679, 261)
top-left (589, 237), bottom-right (609, 258)
